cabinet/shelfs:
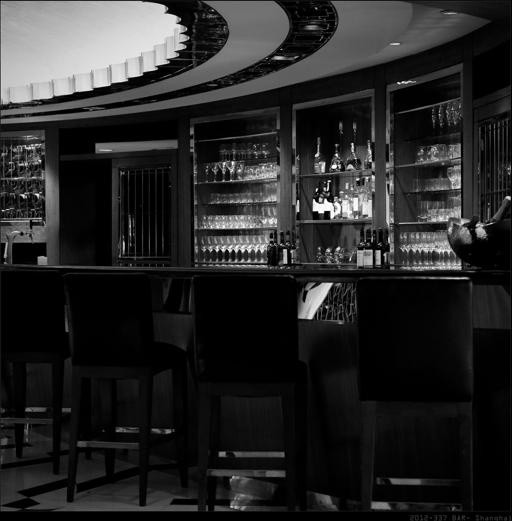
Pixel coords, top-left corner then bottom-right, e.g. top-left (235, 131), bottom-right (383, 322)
top-left (293, 93), bottom-right (378, 273)
top-left (0, 129), bottom-right (60, 265)
top-left (383, 65), bottom-right (468, 273)
top-left (191, 107), bottom-right (284, 271)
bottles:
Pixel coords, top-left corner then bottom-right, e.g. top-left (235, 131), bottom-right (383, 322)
top-left (354, 227), bottom-right (393, 269)
top-left (307, 122), bottom-right (375, 219)
top-left (266, 228), bottom-right (303, 268)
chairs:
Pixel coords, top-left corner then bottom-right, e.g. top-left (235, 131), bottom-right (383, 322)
top-left (0, 269), bottom-right (72, 474)
top-left (64, 273), bottom-right (188, 506)
top-left (193, 274), bottom-right (309, 512)
top-left (357, 277), bottom-right (474, 511)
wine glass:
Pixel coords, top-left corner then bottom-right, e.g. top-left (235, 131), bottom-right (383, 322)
top-left (447, 166), bottom-right (465, 189)
top-left (197, 141), bottom-right (279, 229)
top-left (200, 233), bottom-right (267, 263)
top-left (400, 227), bottom-right (460, 270)
top-left (416, 194), bottom-right (463, 223)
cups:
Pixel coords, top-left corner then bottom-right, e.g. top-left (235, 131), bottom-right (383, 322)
top-left (412, 142), bottom-right (463, 163)
top-left (408, 178), bottom-right (448, 193)
